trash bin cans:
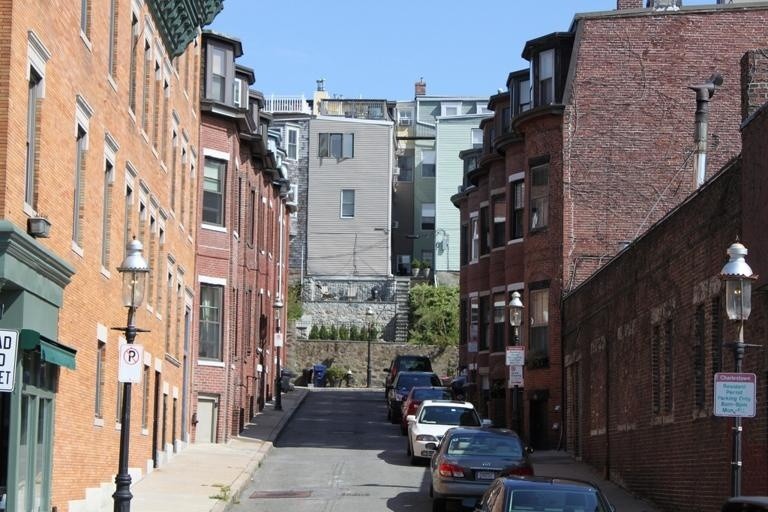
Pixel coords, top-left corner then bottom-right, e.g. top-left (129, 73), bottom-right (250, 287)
top-left (280, 368), bottom-right (291, 393)
top-left (302, 368), bottom-right (313, 386)
top-left (451, 379), bottom-right (466, 401)
top-left (313, 365), bottom-right (327, 387)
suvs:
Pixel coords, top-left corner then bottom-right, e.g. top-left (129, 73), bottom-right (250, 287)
top-left (383, 354), bottom-right (432, 395)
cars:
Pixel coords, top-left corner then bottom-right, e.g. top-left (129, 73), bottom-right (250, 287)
top-left (405, 398), bottom-right (495, 466)
top-left (477, 473), bottom-right (616, 512)
top-left (721, 495), bottom-right (766, 511)
top-left (425, 421), bottom-right (537, 512)
top-left (402, 384), bottom-right (451, 438)
top-left (385, 371), bottom-right (440, 425)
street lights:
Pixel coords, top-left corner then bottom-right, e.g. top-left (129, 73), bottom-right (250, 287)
top-left (106, 236), bottom-right (152, 512)
top-left (270, 291), bottom-right (286, 411)
top-left (504, 290), bottom-right (531, 457)
top-left (716, 234), bottom-right (761, 498)
top-left (366, 303), bottom-right (374, 388)
